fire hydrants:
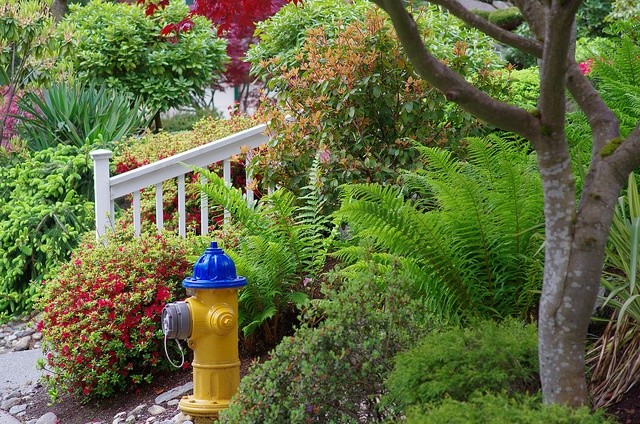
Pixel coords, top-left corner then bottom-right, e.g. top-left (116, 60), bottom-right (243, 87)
top-left (161, 241), bottom-right (247, 424)
